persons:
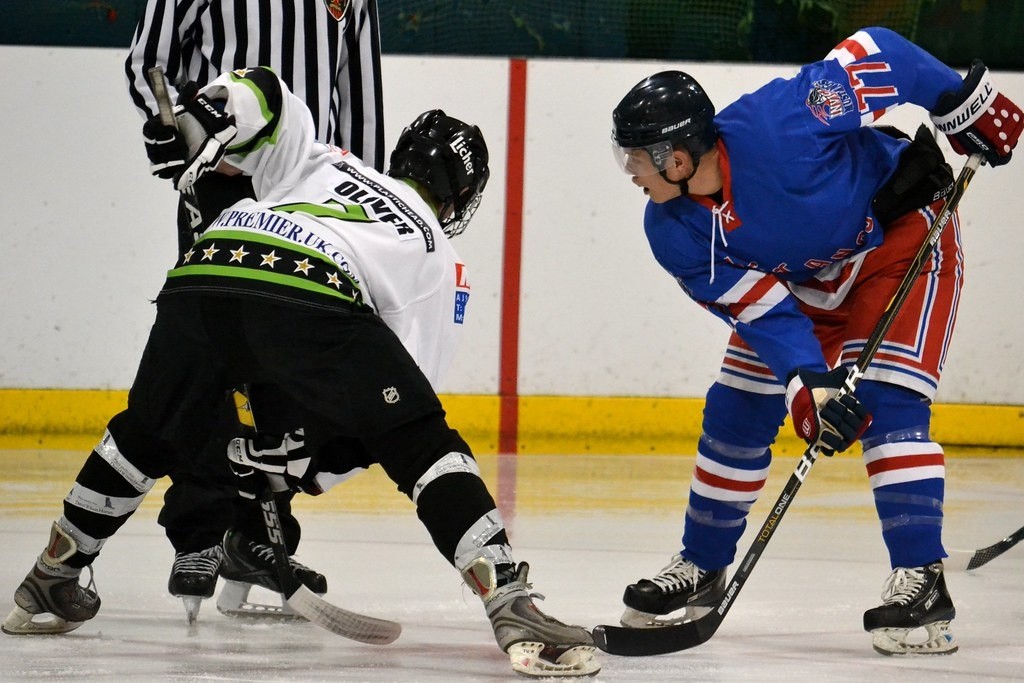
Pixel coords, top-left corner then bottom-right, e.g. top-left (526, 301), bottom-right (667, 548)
top-left (609, 24), bottom-right (1024, 658)
top-left (124, 0), bottom-right (385, 625)
top-left (0, 65), bottom-right (603, 678)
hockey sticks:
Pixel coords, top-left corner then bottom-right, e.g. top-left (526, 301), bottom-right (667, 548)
top-left (148, 65), bottom-right (401, 646)
top-left (593, 148), bottom-right (1024, 657)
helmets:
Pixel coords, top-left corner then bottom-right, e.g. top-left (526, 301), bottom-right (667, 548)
top-left (612, 70), bottom-right (718, 161)
top-left (390, 109), bottom-right (490, 239)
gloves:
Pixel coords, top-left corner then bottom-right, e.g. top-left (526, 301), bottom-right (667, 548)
top-left (144, 80), bottom-right (236, 192)
top-left (929, 59), bottom-right (1024, 168)
top-left (783, 366), bottom-right (873, 458)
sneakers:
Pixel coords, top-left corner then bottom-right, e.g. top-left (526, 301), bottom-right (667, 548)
top-left (462, 560), bottom-right (602, 677)
top-left (863, 559), bottom-right (958, 658)
top-left (622, 551), bottom-right (726, 627)
top-left (2, 521), bottom-right (101, 634)
top-left (170, 542), bottom-right (223, 623)
top-left (216, 521), bottom-right (328, 618)
